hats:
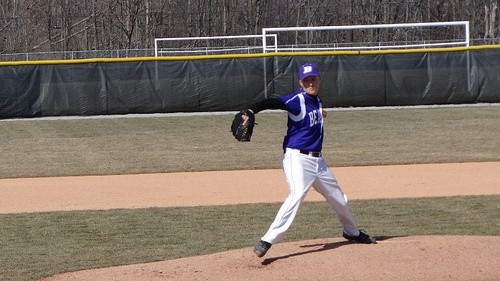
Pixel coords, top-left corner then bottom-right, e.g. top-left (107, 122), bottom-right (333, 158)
top-left (298, 62), bottom-right (320, 81)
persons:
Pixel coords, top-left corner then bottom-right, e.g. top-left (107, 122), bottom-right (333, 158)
top-left (240, 64), bottom-right (377, 258)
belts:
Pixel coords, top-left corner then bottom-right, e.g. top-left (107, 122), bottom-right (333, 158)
top-left (300, 150), bottom-right (319, 157)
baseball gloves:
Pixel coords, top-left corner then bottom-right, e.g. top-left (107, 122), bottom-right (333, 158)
top-left (230, 109), bottom-right (258, 142)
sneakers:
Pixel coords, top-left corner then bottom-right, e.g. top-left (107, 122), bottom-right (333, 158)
top-left (343, 230), bottom-right (376, 243)
top-left (253, 240), bottom-right (272, 257)
